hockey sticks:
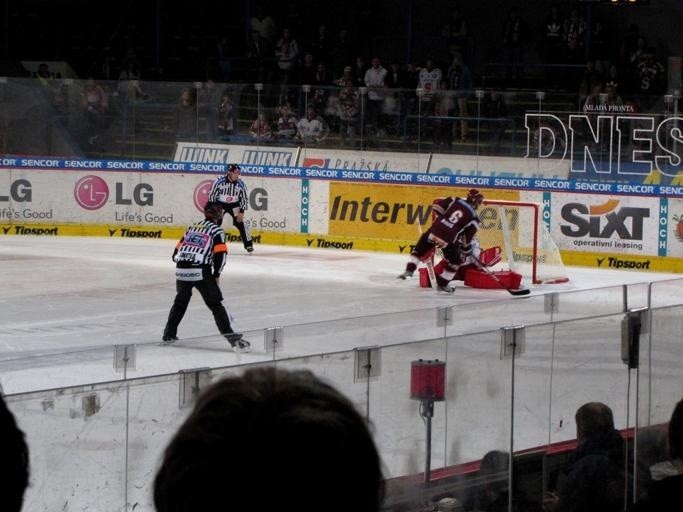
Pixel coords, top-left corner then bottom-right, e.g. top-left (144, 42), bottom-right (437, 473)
top-left (468, 254), bottom-right (530, 296)
top-left (414, 217), bottom-right (455, 291)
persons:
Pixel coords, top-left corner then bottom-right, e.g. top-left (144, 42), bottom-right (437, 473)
top-left (556, 121), bottom-right (577, 150)
top-left (605, 38), bottom-right (665, 112)
top-left (163, 201), bottom-right (251, 347)
top-left (466, 451), bottom-right (528, 512)
top-left (178, 15), bottom-right (513, 156)
top-left (650, 398), bottom-right (682, 512)
top-left (421, 234), bottom-right (521, 290)
top-left (208, 164), bottom-right (253, 251)
top-left (530, 119), bottom-right (561, 150)
top-left (151, 367), bottom-right (386, 512)
top-left (542, 402), bottom-right (650, 511)
top-left (581, 83), bottom-right (637, 147)
top-left (30, 63), bottom-right (150, 158)
top-left (399, 188), bottom-right (483, 292)
top-left (0, 381), bottom-right (30, 510)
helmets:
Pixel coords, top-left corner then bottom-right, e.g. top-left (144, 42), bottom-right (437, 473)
top-left (228, 164), bottom-right (240, 175)
top-left (467, 189), bottom-right (483, 210)
top-left (204, 202), bottom-right (226, 225)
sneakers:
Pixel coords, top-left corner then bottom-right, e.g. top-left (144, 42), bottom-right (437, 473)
top-left (246, 246), bottom-right (253, 252)
top-left (231, 339), bottom-right (251, 349)
top-left (397, 271), bottom-right (413, 279)
top-left (439, 285), bottom-right (455, 292)
top-left (163, 336), bottom-right (179, 341)
